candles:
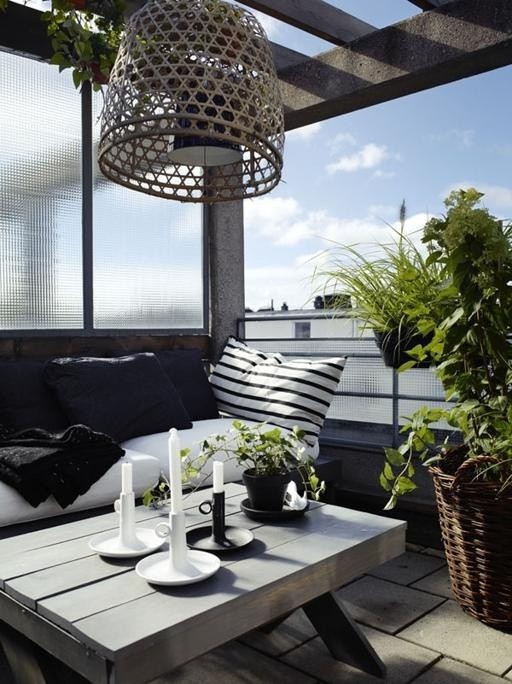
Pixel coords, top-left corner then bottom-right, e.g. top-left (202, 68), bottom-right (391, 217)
top-left (121, 427), bottom-right (224, 511)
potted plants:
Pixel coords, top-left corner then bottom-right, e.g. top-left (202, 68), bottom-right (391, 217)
top-left (379, 188), bottom-right (512, 635)
top-left (46, 8), bottom-right (148, 91)
top-left (138, 419), bottom-right (324, 522)
top-left (287, 208), bottom-right (450, 369)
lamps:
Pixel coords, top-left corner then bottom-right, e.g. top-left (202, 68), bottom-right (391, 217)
top-left (98, 0), bottom-right (286, 205)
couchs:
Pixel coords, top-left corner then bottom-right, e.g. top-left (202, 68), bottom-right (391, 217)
top-left (1, 348), bottom-right (345, 506)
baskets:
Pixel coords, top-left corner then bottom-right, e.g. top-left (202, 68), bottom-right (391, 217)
top-left (428, 443), bottom-right (512, 630)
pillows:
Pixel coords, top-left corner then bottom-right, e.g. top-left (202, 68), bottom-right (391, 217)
top-left (42, 351), bottom-right (193, 444)
